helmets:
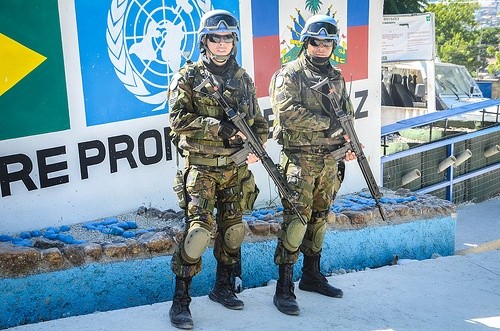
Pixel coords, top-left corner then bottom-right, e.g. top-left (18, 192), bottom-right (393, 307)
top-left (198, 9), bottom-right (238, 37)
top-left (301, 14), bottom-right (339, 41)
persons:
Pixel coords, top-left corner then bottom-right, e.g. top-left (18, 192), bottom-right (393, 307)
top-left (268, 15), bottom-right (358, 316)
top-left (168, 9), bottom-right (269, 330)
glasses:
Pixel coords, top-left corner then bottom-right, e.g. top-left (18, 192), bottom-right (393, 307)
top-left (306, 21), bottom-right (338, 38)
top-left (208, 35), bottom-right (234, 43)
top-left (203, 15), bottom-right (238, 30)
top-left (309, 38), bottom-right (333, 47)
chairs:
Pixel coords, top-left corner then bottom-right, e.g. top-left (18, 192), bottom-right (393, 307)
top-left (382, 74), bottom-right (412, 107)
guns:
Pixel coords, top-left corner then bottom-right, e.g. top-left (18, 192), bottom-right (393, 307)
top-left (193, 75), bottom-right (307, 227)
top-left (310, 75), bottom-right (387, 223)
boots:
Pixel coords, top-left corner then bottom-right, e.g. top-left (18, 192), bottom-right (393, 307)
top-left (169, 275), bottom-right (194, 329)
top-left (273, 263), bottom-right (300, 315)
top-left (207, 261), bottom-right (244, 309)
top-left (298, 254), bottom-right (344, 298)
